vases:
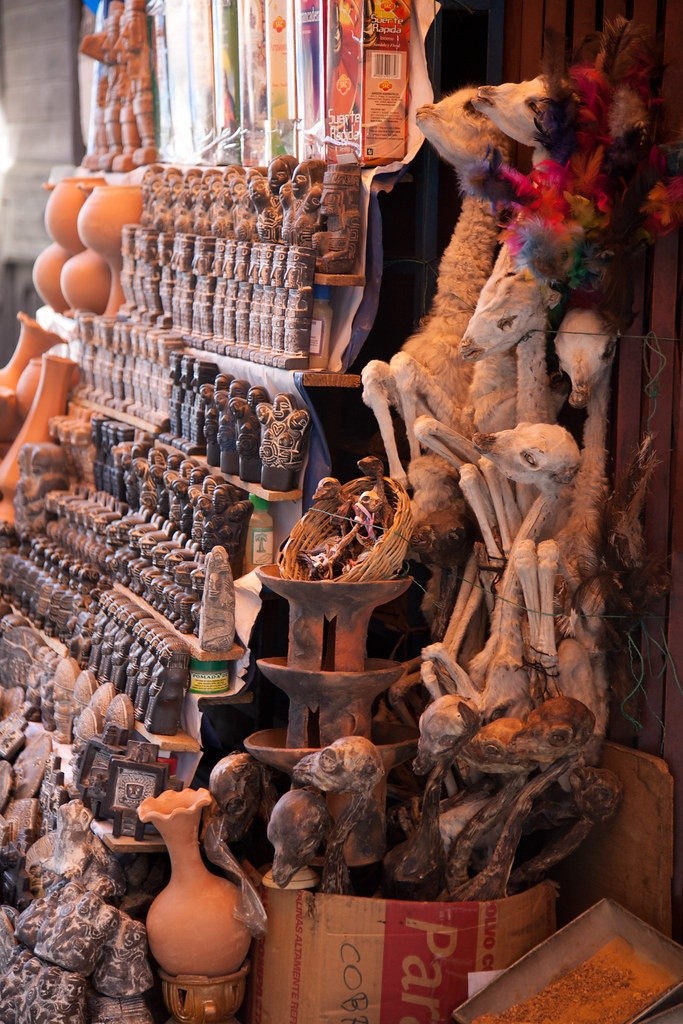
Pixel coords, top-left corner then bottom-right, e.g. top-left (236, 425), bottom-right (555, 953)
top-left (76, 186), bottom-right (146, 317)
top-left (137, 789), bottom-right (255, 978)
top-left (0, 312), bottom-right (69, 391)
top-left (0, 356), bottom-right (77, 529)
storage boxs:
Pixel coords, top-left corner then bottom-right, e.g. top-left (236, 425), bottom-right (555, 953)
top-left (245, 863), bottom-right (560, 1024)
top-left (151, 1), bottom-right (409, 167)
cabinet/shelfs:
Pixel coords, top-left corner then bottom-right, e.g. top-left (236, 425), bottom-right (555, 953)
top-left (16, 170), bottom-right (415, 853)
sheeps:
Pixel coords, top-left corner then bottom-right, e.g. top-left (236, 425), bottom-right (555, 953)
top-left (358, 66), bottom-right (645, 742)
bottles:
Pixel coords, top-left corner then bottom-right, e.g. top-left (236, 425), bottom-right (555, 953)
top-left (135, 783), bottom-right (253, 977)
top-left (309, 287), bottom-right (334, 369)
top-left (242, 493), bottom-right (274, 576)
